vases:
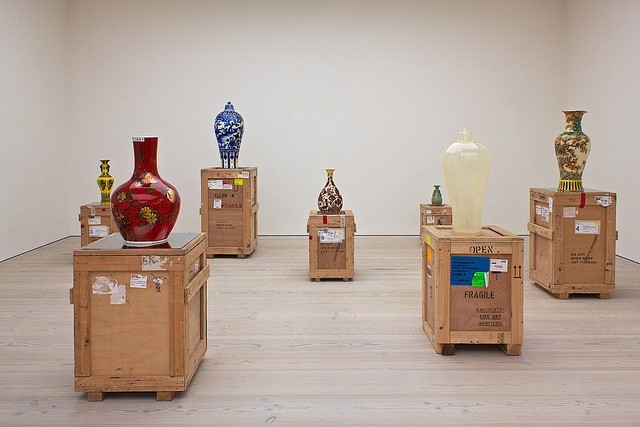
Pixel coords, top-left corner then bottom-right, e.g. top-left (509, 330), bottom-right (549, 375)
top-left (214, 101), bottom-right (244, 168)
top-left (97, 159), bottom-right (114, 205)
top-left (318, 167), bottom-right (343, 214)
top-left (555, 110), bottom-right (591, 191)
top-left (111, 135), bottom-right (180, 247)
top-left (431, 184), bottom-right (442, 205)
top-left (443, 128), bottom-right (489, 235)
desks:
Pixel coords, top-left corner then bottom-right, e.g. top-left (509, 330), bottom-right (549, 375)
top-left (419, 226), bottom-right (524, 357)
top-left (78, 200), bottom-right (115, 246)
top-left (306, 211), bottom-right (355, 282)
top-left (529, 188), bottom-right (618, 300)
top-left (69, 231), bottom-right (210, 401)
top-left (419, 203), bottom-right (452, 243)
top-left (199, 167), bottom-right (259, 258)
